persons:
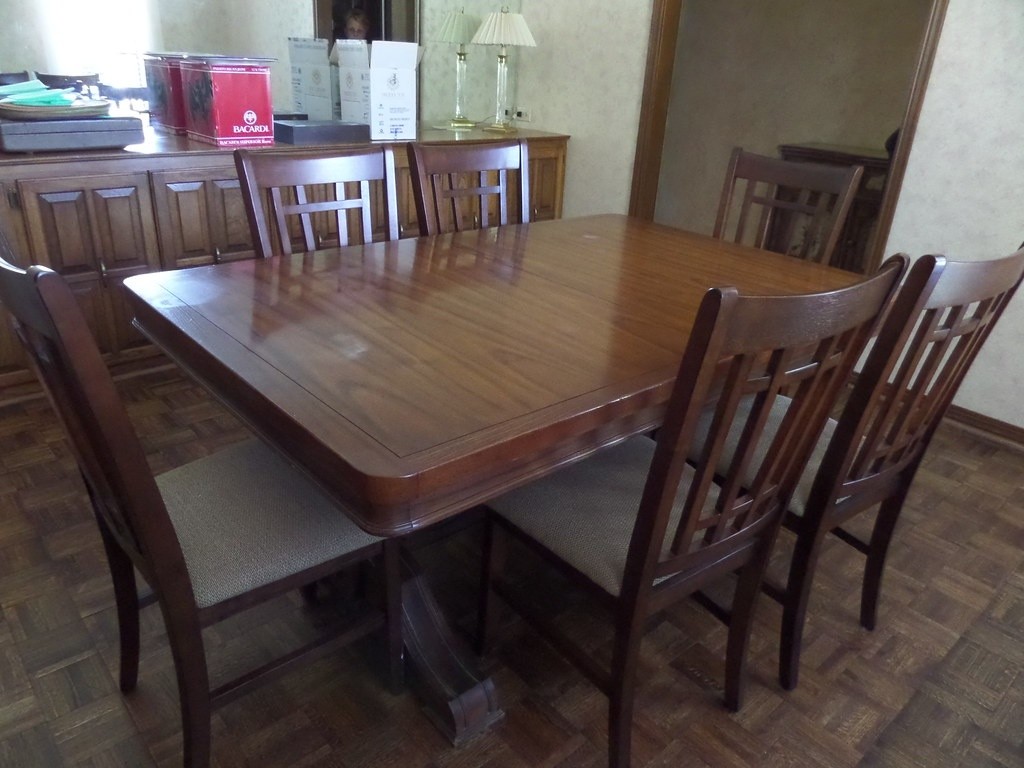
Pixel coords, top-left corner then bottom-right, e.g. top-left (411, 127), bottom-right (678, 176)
top-left (345, 7), bottom-right (370, 42)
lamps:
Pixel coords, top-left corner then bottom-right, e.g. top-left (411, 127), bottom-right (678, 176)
top-left (430, 6), bottom-right (493, 129)
top-left (470, 5), bottom-right (536, 134)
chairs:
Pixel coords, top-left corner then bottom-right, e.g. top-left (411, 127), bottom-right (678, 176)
top-left (472, 252), bottom-right (911, 767)
top-left (711, 147), bottom-right (864, 396)
top-left (651, 242), bottom-right (1024, 691)
top-left (95, 80), bottom-right (149, 110)
top-left (406, 138), bottom-right (532, 237)
top-left (233, 143), bottom-right (400, 259)
top-left (0, 233), bottom-right (405, 768)
top-left (34, 71), bottom-right (101, 89)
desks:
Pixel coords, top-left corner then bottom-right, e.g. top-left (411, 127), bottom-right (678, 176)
top-left (122, 213), bottom-right (872, 750)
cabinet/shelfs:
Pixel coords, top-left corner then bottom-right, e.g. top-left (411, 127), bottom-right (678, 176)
top-left (765, 142), bottom-right (891, 271)
top-left (0, 124), bottom-right (572, 410)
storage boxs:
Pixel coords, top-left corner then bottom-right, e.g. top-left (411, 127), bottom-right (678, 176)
top-left (282, 36), bottom-right (341, 121)
top-left (337, 38), bottom-right (426, 141)
top-left (143, 52), bottom-right (225, 136)
top-left (179, 59), bottom-right (274, 148)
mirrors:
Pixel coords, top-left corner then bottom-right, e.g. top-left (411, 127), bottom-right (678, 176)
top-left (0, 0), bottom-right (524, 137)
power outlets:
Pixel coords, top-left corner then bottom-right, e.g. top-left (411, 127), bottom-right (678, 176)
top-left (504, 105), bottom-right (513, 120)
top-left (513, 105), bottom-right (532, 122)
